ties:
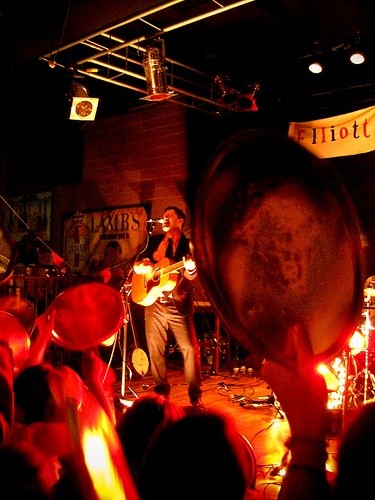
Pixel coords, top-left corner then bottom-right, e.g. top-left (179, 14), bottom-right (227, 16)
top-left (165, 239), bottom-right (174, 257)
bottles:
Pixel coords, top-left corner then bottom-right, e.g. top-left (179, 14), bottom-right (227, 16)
top-left (169, 345), bottom-right (174, 354)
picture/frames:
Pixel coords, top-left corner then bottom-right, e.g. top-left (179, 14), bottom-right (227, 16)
top-left (3, 187), bottom-right (56, 243)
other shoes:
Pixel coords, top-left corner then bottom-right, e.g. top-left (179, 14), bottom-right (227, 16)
top-left (190, 399), bottom-right (204, 411)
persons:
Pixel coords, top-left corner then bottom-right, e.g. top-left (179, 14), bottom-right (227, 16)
top-left (6, 235), bottom-right (39, 273)
top-left (0, 307), bottom-right (375, 500)
top-left (130, 207), bottom-right (204, 410)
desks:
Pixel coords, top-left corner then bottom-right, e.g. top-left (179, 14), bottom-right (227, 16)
top-left (191, 303), bottom-right (234, 375)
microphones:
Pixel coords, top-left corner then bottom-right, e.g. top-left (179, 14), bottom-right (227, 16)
top-left (147, 217), bottom-right (164, 224)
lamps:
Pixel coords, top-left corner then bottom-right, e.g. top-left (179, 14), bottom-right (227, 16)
top-left (137, 37), bottom-right (178, 102)
top-left (211, 71), bottom-right (242, 109)
top-left (234, 77), bottom-right (263, 114)
top-left (64, 80), bottom-right (99, 121)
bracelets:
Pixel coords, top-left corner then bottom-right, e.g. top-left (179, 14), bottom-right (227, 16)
top-left (289, 463), bottom-right (325, 476)
top-left (284, 435), bottom-right (326, 451)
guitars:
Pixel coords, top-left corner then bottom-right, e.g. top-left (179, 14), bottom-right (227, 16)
top-left (131, 257), bottom-right (193, 307)
